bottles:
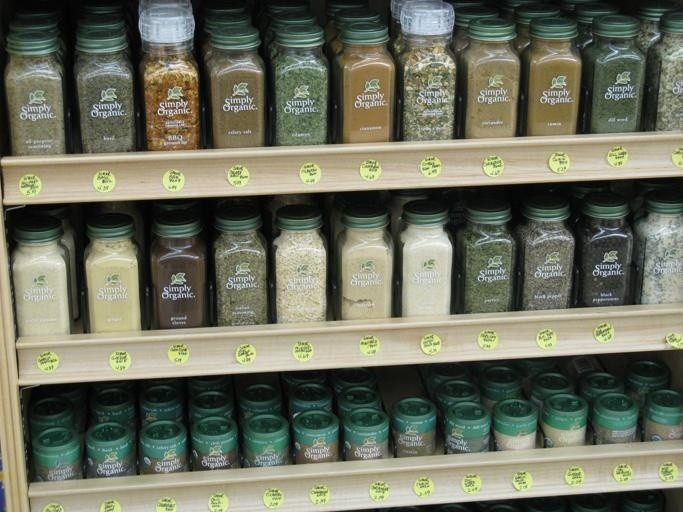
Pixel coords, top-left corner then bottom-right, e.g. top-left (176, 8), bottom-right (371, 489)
top-left (2, 0), bottom-right (681, 158)
top-left (9, 182), bottom-right (682, 338)
top-left (25, 355), bottom-right (681, 482)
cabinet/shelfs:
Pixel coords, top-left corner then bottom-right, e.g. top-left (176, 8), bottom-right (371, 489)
top-left (1, 2), bottom-right (683, 512)
